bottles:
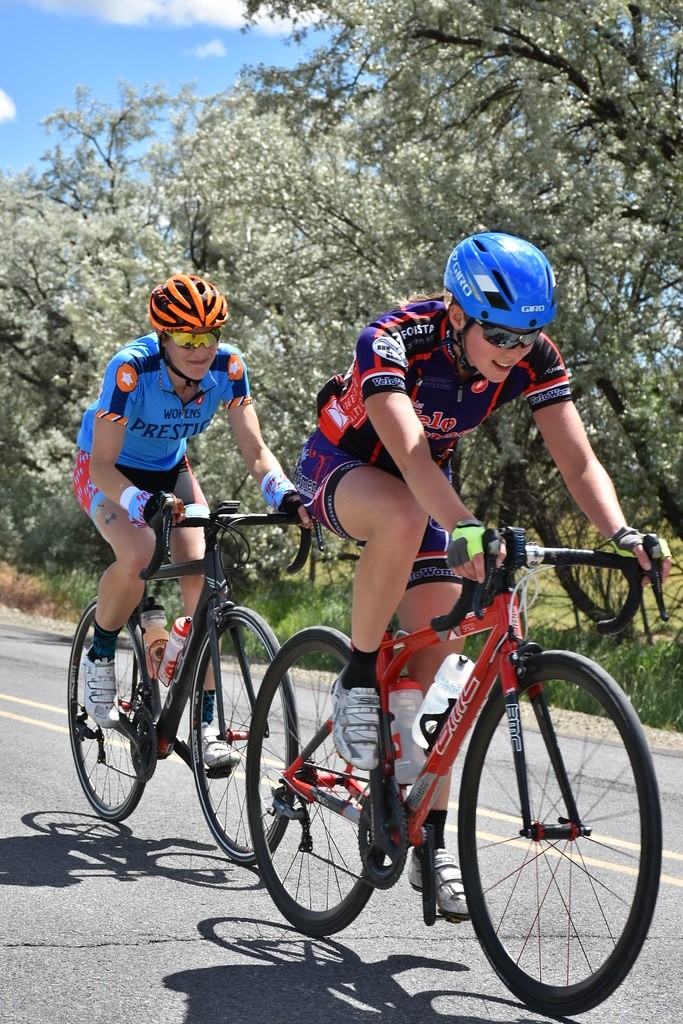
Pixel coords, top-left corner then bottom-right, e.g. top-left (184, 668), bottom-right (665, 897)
top-left (412, 654), bottom-right (474, 749)
top-left (157, 616), bottom-right (193, 687)
top-left (141, 596), bottom-right (169, 679)
top-left (388, 675), bottom-right (429, 785)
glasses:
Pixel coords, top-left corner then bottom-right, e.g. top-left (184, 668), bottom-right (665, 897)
top-left (163, 324), bottom-right (225, 349)
top-left (472, 317), bottom-right (541, 349)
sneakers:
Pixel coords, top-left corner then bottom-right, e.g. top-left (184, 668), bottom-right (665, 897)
top-left (187, 721), bottom-right (240, 768)
top-left (330, 662), bottom-right (381, 771)
top-left (408, 843), bottom-right (470, 915)
top-left (83, 643), bottom-right (120, 728)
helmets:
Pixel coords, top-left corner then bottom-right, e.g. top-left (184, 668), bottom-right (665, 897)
top-left (149, 274), bottom-right (229, 332)
top-left (444, 232), bottom-right (558, 330)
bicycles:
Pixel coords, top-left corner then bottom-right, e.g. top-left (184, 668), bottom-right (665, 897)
top-left (245, 526), bottom-right (669, 1016)
top-left (65, 499), bottom-right (325, 865)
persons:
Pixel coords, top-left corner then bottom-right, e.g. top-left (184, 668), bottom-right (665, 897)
top-left (73, 275), bottom-right (315, 765)
top-left (289, 233), bottom-right (671, 918)
